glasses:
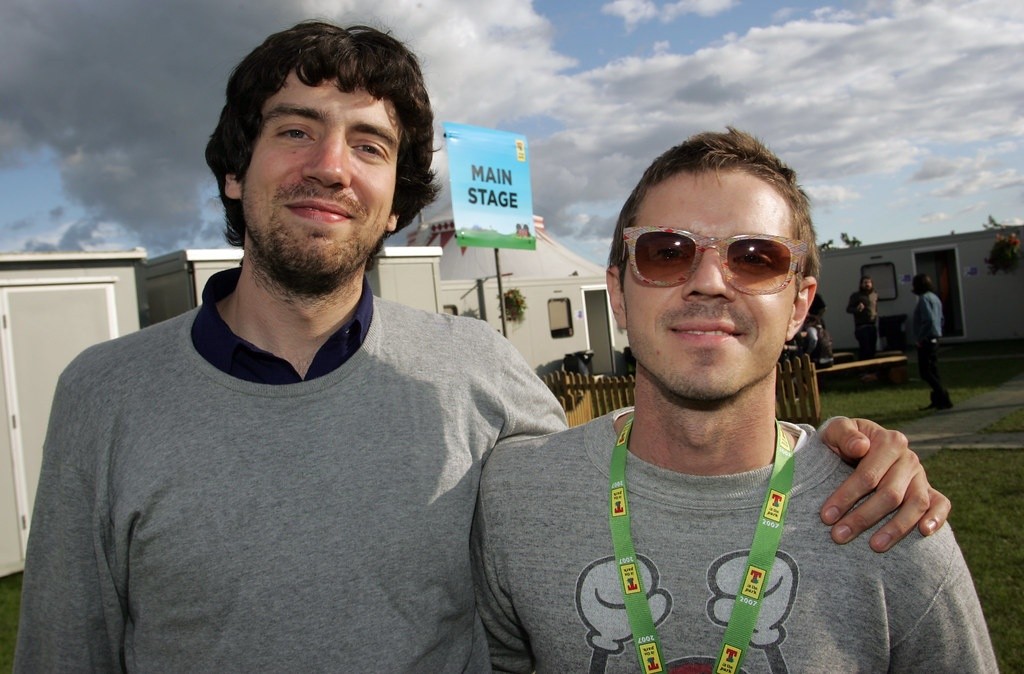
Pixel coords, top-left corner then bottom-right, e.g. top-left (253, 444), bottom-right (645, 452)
top-left (620, 226), bottom-right (805, 295)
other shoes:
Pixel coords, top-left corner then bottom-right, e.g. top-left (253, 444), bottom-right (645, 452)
top-left (918, 401), bottom-right (954, 409)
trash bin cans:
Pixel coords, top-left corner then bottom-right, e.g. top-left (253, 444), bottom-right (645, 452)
top-left (878, 313), bottom-right (908, 353)
top-left (562, 350), bottom-right (594, 376)
top-left (624, 347), bottom-right (636, 376)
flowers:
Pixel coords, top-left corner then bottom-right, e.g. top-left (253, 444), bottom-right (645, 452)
top-left (495, 286), bottom-right (530, 325)
top-left (987, 230), bottom-right (1024, 275)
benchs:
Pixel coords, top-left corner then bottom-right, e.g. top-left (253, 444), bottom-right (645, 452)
top-left (816, 354), bottom-right (910, 390)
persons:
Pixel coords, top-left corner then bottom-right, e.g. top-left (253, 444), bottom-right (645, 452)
top-left (787, 274), bottom-right (881, 382)
top-left (900, 273), bottom-right (954, 411)
top-left (12, 20), bottom-right (950, 674)
top-left (464, 126), bottom-right (999, 674)
